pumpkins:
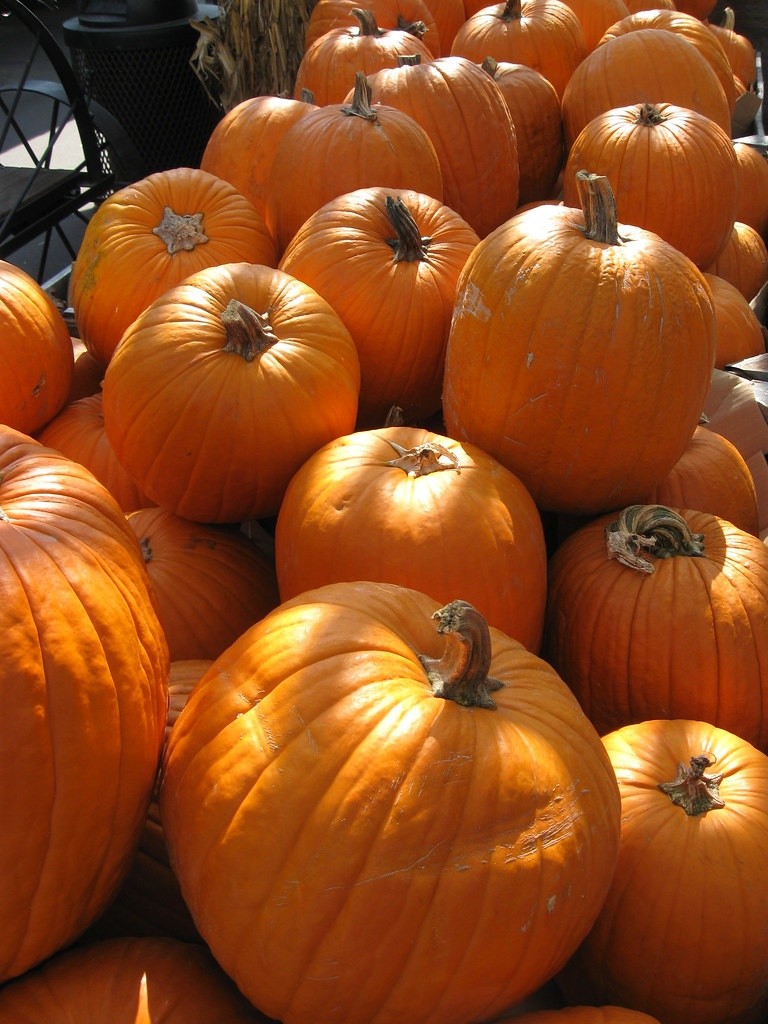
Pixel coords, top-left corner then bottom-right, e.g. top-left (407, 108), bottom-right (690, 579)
top-left (0, 0), bottom-right (768, 1024)
